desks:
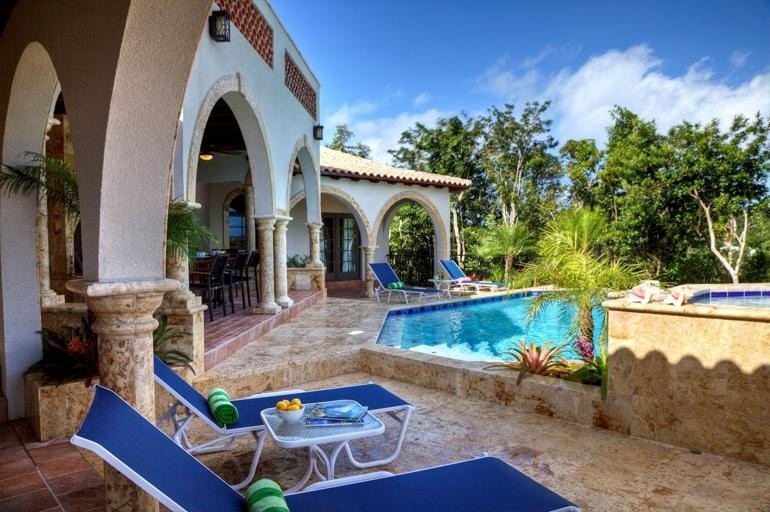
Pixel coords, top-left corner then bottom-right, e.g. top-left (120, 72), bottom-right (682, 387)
top-left (230, 398), bottom-right (385, 494)
top-left (427, 278), bottom-right (458, 300)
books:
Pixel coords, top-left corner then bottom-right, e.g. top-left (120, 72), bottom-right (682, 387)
top-left (304, 403), bottom-right (368, 421)
top-left (305, 418), bottom-right (363, 427)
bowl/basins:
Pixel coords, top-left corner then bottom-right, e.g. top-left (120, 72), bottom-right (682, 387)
top-left (276, 404), bottom-right (305, 423)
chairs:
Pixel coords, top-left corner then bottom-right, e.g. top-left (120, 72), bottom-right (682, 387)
top-left (55, 400), bottom-right (581, 511)
top-left (151, 356), bottom-right (413, 471)
top-left (253, 248), bottom-right (262, 306)
top-left (231, 249), bottom-right (251, 313)
top-left (367, 262), bottom-right (443, 303)
top-left (437, 260), bottom-right (503, 296)
top-left (197, 253), bottom-right (226, 320)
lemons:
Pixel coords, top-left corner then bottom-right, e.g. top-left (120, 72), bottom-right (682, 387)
top-left (276, 398), bottom-right (301, 411)
